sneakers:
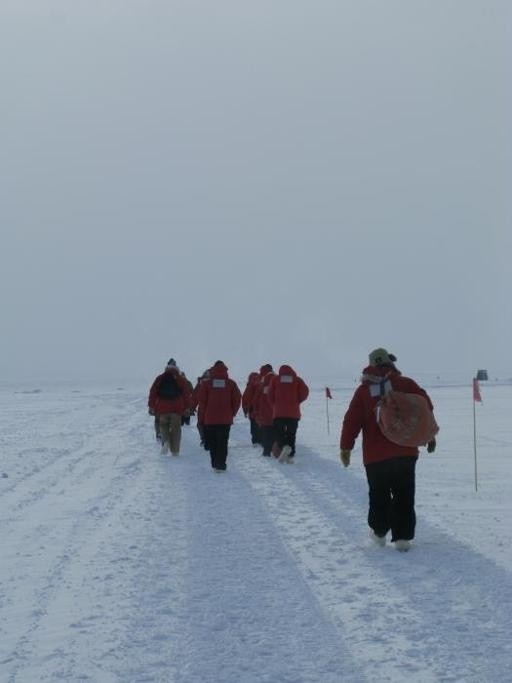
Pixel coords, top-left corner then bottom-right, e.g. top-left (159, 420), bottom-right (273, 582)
top-left (368, 529), bottom-right (386, 548)
top-left (394, 538), bottom-right (411, 552)
top-left (277, 444), bottom-right (295, 464)
top-left (160, 442), bottom-right (170, 454)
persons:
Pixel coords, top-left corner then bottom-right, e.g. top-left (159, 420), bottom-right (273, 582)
top-left (340, 348), bottom-right (436, 551)
top-left (149, 359), bottom-right (309, 473)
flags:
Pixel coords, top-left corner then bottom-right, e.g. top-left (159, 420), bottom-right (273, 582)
top-left (473, 378), bottom-right (481, 401)
top-left (326, 388), bottom-right (332, 399)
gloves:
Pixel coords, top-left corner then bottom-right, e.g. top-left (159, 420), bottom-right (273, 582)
top-left (424, 437), bottom-right (436, 453)
top-left (340, 448), bottom-right (351, 467)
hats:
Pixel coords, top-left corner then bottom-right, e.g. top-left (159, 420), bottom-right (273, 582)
top-left (368, 347), bottom-right (396, 367)
